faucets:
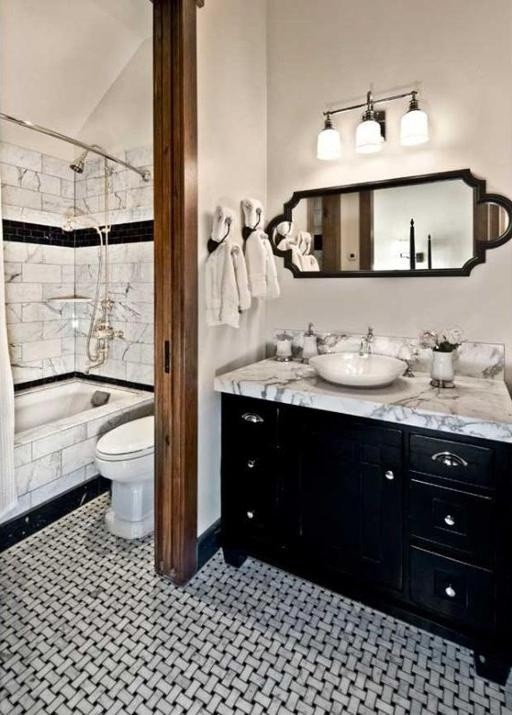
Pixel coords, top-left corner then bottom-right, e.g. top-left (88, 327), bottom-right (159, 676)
top-left (86, 359), bottom-right (103, 376)
top-left (359, 328), bottom-right (375, 357)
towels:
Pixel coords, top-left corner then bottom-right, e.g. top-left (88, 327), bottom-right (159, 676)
top-left (278, 219), bottom-right (324, 271)
top-left (197, 197), bottom-right (281, 326)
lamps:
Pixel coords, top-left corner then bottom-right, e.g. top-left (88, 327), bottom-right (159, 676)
top-left (316, 87), bottom-right (429, 162)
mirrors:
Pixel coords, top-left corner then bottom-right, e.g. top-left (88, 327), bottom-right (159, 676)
top-left (265, 168), bottom-right (511, 277)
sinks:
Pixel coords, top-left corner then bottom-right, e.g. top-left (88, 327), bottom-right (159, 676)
top-left (308, 352), bottom-right (408, 388)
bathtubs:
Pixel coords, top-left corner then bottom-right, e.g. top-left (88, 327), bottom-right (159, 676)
top-left (15, 375), bottom-right (155, 450)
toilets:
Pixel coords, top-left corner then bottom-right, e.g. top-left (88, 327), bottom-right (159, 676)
top-left (95, 413), bottom-right (155, 541)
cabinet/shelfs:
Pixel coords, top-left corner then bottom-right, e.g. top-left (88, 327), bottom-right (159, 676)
top-left (281, 402), bottom-right (405, 625)
top-left (405, 424), bottom-right (511, 687)
top-left (220, 394), bottom-right (281, 570)
